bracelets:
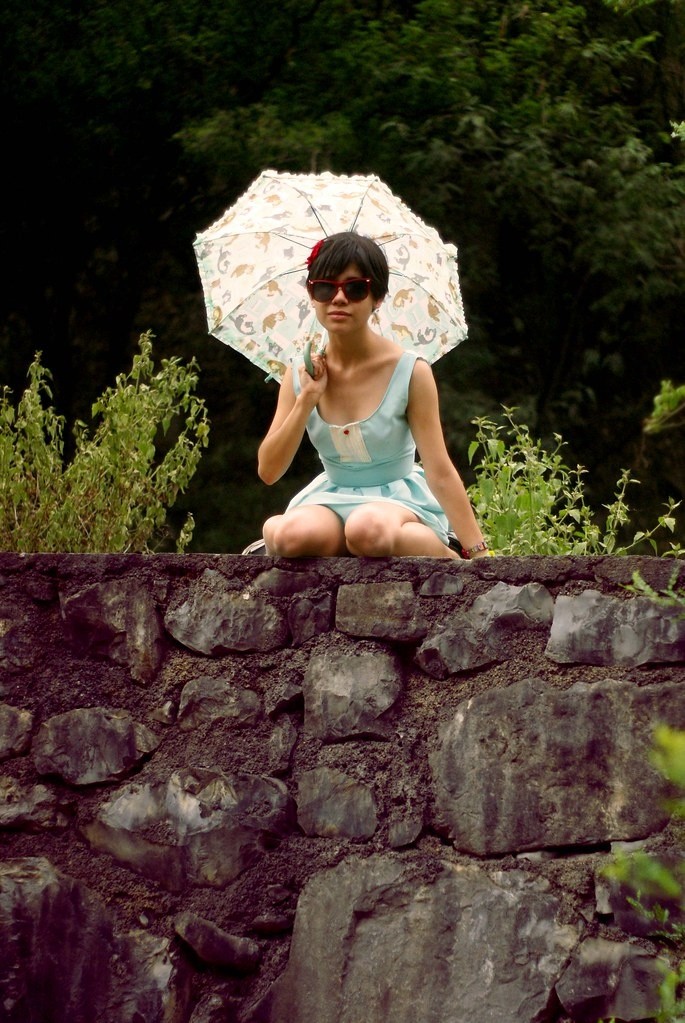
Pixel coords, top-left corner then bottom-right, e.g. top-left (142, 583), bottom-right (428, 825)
top-left (462, 541), bottom-right (488, 559)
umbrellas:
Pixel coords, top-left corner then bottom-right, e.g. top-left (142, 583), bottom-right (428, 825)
top-left (193, 170), bottom-right (468, 385)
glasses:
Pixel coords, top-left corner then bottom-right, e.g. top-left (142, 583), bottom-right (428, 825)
top-left (307, 276), bottom-right (372, 302)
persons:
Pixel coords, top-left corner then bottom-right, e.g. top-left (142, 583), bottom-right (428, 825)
top-left (257, 232), bottom-right (490, 559)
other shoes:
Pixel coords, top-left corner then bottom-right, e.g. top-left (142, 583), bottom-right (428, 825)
top-left (242, 539), bottom-right (266, 555)
top-left (447, 529), bottom-right (469, 558)
